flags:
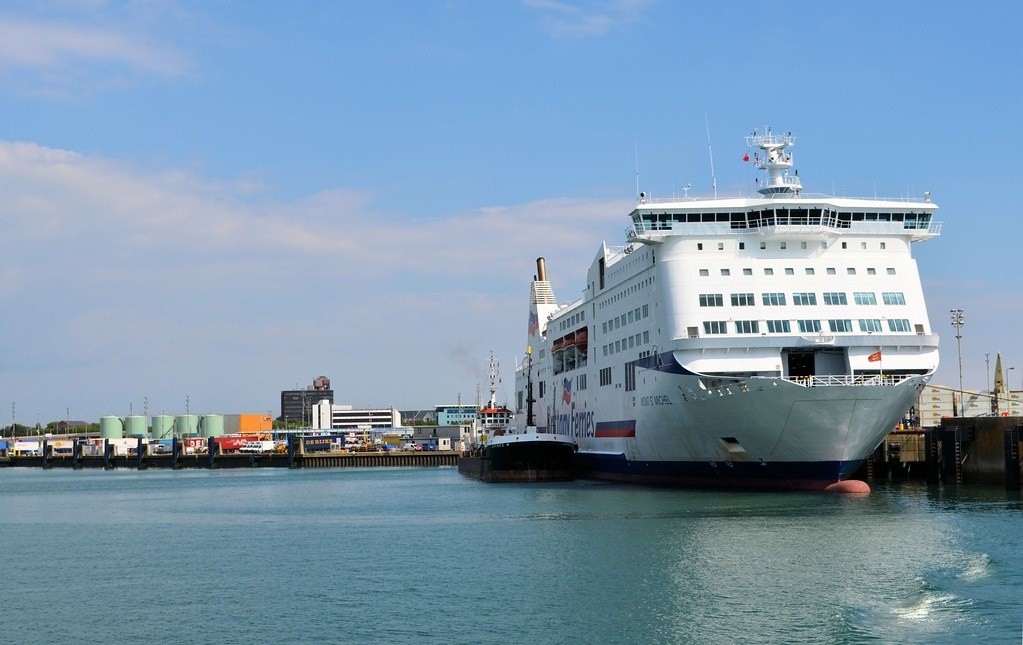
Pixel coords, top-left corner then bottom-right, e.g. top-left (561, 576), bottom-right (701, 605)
top-left (742, 152), bottom-right (750, 162)
top-left (868, 351), bottom-right (882, 361)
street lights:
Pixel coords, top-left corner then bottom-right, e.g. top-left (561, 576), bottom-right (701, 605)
top-left (983, 352), bottom-right (991, 415)
top-left (1006, 366), bottom-right (1014, 415)
top-left (950, 307), bottom-right (966, 417)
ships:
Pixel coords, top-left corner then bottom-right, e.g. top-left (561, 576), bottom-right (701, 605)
top-left (512, 113), bottom-right (942, 494)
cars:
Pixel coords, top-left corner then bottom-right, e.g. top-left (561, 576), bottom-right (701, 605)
top-left (3, 438), bottom-right (474, 465)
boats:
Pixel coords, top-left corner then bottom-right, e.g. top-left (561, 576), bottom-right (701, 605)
top-left (456, 349), bottom-right (580, 483)
